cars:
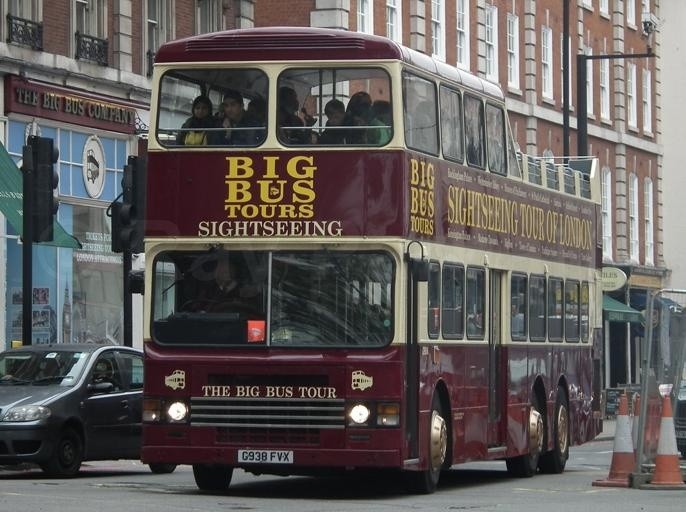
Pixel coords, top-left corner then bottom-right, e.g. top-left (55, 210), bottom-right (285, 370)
top-left (0, 344), bottom-right (177, 478)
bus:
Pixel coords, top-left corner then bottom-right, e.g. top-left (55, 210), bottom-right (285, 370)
top-left (127, 27), bottom-right (604, 494)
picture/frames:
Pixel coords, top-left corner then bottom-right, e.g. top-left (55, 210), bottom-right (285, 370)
top-left (32, 287), bottom-right (50, 306)
top-left (32, 308), bottom-right (50, 329)
top-left (32, 332), bottom-right (50, 345)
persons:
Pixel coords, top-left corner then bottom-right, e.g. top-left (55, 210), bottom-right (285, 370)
top-left (318, 91), bottom-right (393, 146)
top-left (175, 89), bottom-right (267, 146)
top-left (190, 257), bottom-right (252, 313)
top-left (404, 86), bottom-right (505, 171)
top-left (277, 85), bottom-right (316, 146)
top-left (11, 288), bottom-right (50, 344)
top-left (84, 360), bottom-right (115, 391)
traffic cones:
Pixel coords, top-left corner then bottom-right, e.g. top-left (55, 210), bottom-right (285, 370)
top-left (592, 393), bottom-right (686, 489)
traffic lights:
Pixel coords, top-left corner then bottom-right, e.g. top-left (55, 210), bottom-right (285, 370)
top-left (111, 155), bottom-right (140, 253)
top-left (28, 136), bottom-right (60, 241)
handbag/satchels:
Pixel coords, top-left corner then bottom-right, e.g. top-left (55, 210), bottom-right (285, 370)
top-left (183, 130), bottom-right (207, 146)
